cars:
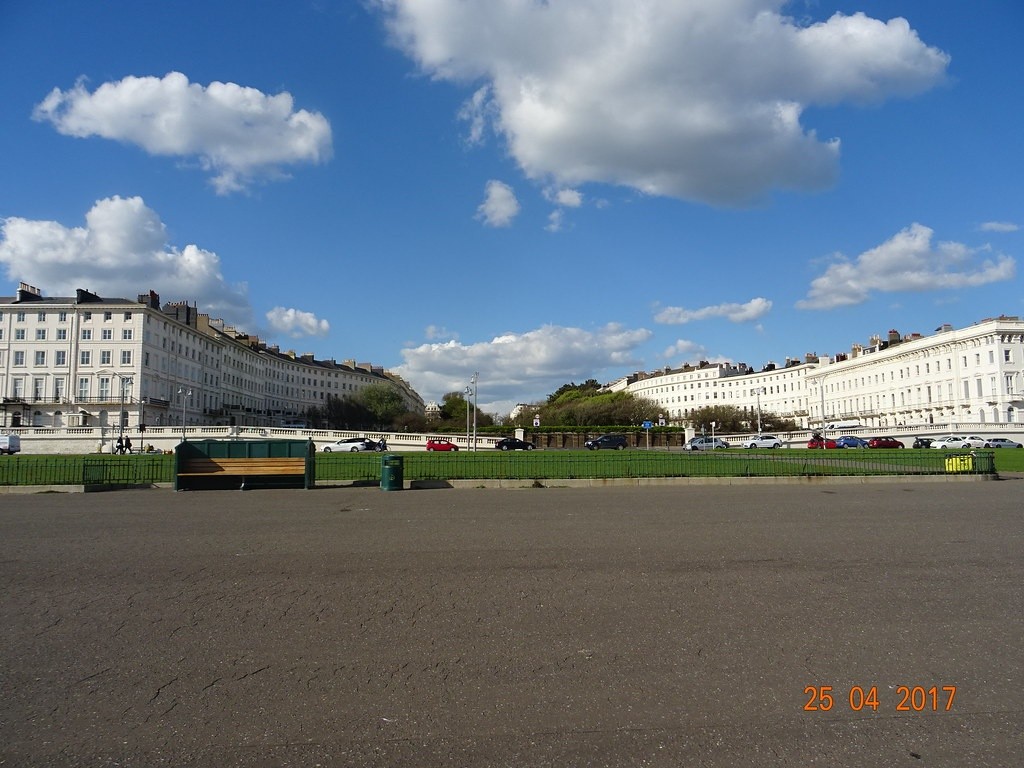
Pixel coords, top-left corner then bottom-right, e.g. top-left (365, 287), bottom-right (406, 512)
top-left (868, 436), bottom-right (905, 450)
top-left (983, 437), bottom-right (1023, 448)
top-left (742, 434), bottom-right (783, 449)
top-left (426, 439), bottom-right (459, 452)
top-left (912, 438), bottom-right (936, 449)
top-left (495, 438), bottom-right (536, 451)
top-left (683, 436), bottom-right (730, 451)
top-left (959, 435), bottom-right (986, 449)
top-left (836, 436), bottom-right (869, 449)
top-left (807, 438), bottom-right (838, 449)
top-left (930, 436), bottom-right (971, 450)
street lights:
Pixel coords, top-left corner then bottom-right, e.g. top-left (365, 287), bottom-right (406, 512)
top-left (111, 371), bottom-right (134, 455)
top-left (178, 385), bottom-right (192, 442)
top-left (470, 371), bottom-right (480, 452)
top-left (753, 385), bottom-right (767, 436)
top-left (140, 400), bottom-right (146, 453)
top-left (465, 385), bottom-right (473, 452)
top-left (812, 373), bottom-right (830, 449)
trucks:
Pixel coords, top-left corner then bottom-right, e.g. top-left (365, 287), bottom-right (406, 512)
top-left (0, 434), bottom-right (21, 455)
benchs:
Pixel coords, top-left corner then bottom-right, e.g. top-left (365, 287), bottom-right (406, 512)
top-left (131, 447), bottom-right (155, 451)
top-left (175, 439), bottom-right (316, 489)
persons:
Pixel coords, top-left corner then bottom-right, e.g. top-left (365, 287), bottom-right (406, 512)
top-left (121, 436), bottom-right (133, 454)
top-left (378, 438), bottom-right (387, 451)
top-left (114, 437), bottom-right (125, 455)
top-left (925, 439), bottom-right (932, 449)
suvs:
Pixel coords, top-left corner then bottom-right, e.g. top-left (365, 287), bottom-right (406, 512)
top-left (584, 434), bottom-right (628, 451)
top-left (324, 438), bottom-right (366, 453)
top-left (346, 438), bottom-right (383, 451)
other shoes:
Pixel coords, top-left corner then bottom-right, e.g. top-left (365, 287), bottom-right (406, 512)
top-left (129, 452), bottom-right (132, 454)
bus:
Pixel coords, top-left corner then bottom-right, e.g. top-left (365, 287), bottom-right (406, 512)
top-left (281, 424), bottom-right (306, 434)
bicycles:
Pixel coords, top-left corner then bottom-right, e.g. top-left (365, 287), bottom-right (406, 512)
top-left (374, 443), bottom-right (391, 452)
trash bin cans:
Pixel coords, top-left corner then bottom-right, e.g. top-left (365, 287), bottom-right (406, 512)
top-left (382, 455), bottom-right (404, 491)
top-left (945, 453), bottom-right (975, 471)
